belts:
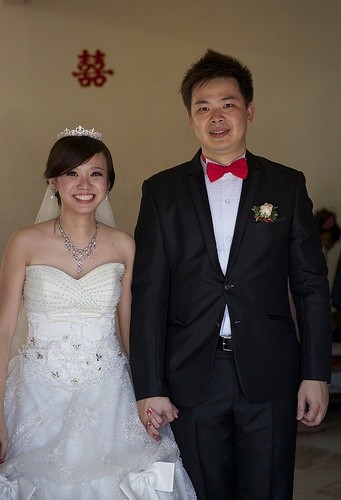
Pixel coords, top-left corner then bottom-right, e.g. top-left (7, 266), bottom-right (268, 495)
top-left (219, 336), bottom-right (234, 352)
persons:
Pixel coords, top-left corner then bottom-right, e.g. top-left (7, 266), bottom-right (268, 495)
top-left (130, 49), bottom-right (341, 500)
top-left (1, 125), bottom-right (198, 500)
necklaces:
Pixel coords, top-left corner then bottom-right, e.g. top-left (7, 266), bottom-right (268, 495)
top-left (53, 214), bottom-right (99, 273)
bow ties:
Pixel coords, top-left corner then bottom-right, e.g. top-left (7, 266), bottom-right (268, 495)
top-left (201, 153), bottom-right (249, 183)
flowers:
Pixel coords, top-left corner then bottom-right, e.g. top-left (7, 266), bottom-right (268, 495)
top-left (251, 202), bottom-right (279, 223)
